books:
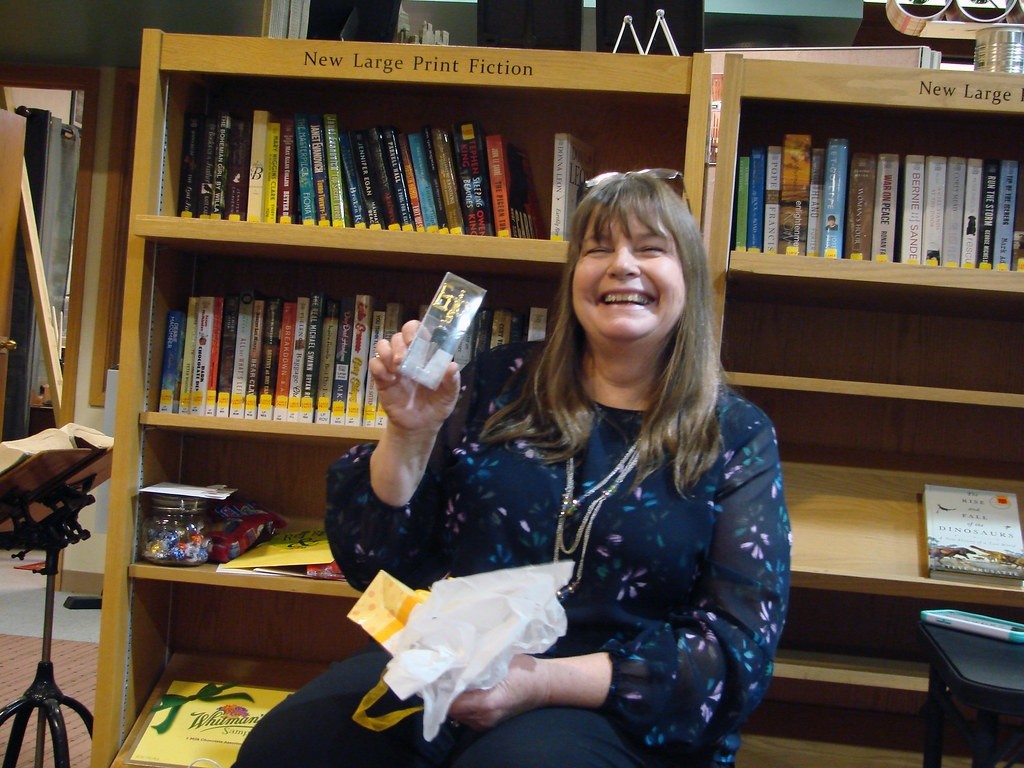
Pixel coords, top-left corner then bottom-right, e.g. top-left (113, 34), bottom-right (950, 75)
top-left (734, 134), bottom-right (1024, 273)
top-left (157, 292), bottom-right (550, 430)
top-left (176, 111), bottom-right (593, 240)
top-left (1, 423), bottom-right (115, 473)
top-left (922, 484), bottom-right (1024, 591)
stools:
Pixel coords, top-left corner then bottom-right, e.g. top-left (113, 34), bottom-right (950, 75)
top-left (919, 616), bottom-right (1024, 768)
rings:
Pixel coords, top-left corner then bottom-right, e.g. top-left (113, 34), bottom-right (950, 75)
top-left (450, 719), bottom-right (460, 727)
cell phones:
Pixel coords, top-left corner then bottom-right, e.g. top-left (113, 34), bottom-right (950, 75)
top-left (920, 610), bottom-right (1024, 645)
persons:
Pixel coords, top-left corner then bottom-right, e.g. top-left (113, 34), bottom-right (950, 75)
top-left (234, 169), bottom-right (791, 768)
top-left (826, 215), bottom-right (838, 231)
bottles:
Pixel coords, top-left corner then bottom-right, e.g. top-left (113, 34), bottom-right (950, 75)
top-left (140, 493), bottom-right (213, 567)
top-left (973, 24), bottom-right (1024, 74)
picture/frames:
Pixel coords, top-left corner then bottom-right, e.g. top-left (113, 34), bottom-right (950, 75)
top-left (67, 90), bottom-right (85, 130)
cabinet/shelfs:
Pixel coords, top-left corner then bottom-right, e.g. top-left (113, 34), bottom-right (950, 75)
top-left (90, 29), bottom-right (1024, 768)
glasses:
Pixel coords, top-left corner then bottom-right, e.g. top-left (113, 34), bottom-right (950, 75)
top-left (576, 169), bottom-right (690, 214)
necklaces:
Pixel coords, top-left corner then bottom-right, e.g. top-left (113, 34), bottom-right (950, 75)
top-left (553, 439), bottom-right (643, 597)
